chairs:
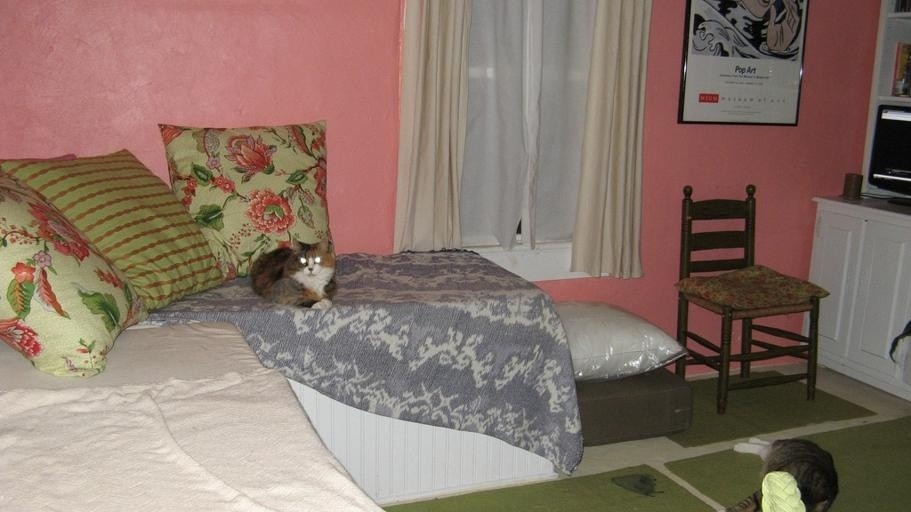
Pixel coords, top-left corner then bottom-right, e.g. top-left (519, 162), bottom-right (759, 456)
top-left (672, 184), bottom-right (830, 415)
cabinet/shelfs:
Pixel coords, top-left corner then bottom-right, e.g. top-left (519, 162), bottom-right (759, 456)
top-left (860, 0), bottom-right (911, 199)
top-left (801, 191), bottom-right (911, 405)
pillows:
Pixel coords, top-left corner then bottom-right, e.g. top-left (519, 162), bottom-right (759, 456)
top-left (156, 118), bottom-right (328, 279)
top-left (555, 297), bottom-right (690, 387)
top-left (1, 164), bottom-right (152, 381)
top-left (2, 146), bottom-right (237, 312)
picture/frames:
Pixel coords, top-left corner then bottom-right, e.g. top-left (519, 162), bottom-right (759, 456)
top-left (676, 1), bottom-right (809, 127)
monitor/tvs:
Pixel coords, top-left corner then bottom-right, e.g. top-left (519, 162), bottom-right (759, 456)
top-left (868, 105), bottom-right (911, 207)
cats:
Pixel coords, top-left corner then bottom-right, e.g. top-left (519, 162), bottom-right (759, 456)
top-left (719, 438), bottom-right (840, 512)
top-left (248, 228), bottom-right (340, 311)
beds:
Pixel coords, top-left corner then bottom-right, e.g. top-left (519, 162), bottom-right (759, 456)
top-left (0, 247), bottom-right (586, 506)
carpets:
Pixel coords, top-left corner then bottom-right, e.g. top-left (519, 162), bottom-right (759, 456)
top-left (664, 414), bottom-right (911, 511)
top-left (382, 461), bottom-right (718, 512)
top-left (662, 369), bottom-right (879, 450)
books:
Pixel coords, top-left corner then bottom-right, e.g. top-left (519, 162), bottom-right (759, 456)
top-left (895, 0), bottom-right (911, 11)
top-left (890, 42), bottom-right (911, 97)
